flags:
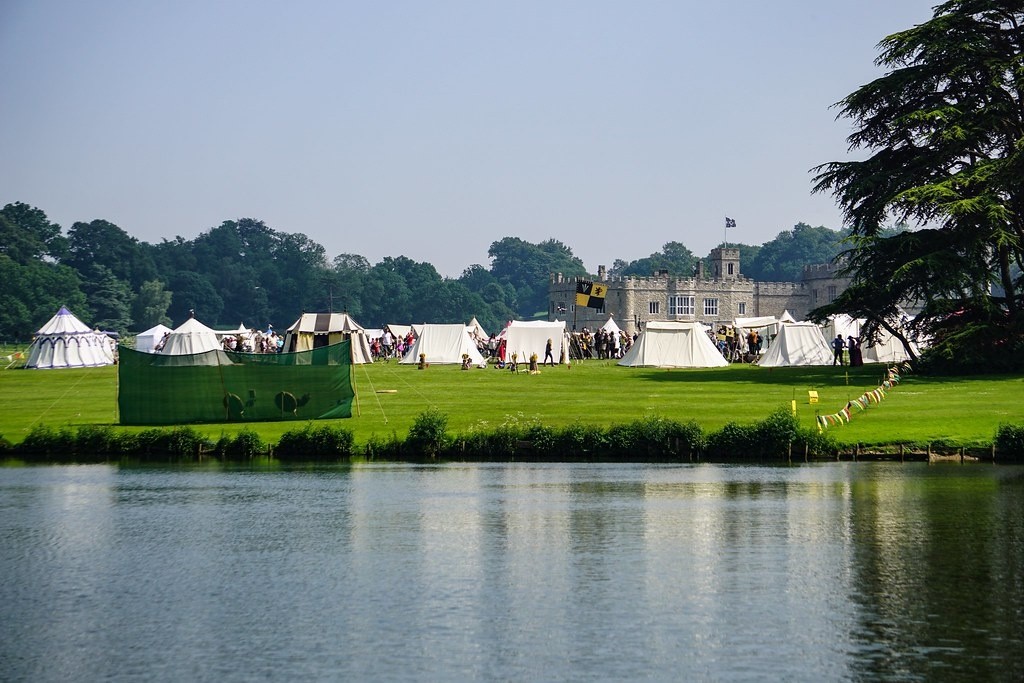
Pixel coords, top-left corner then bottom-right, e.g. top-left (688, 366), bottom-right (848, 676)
top-left (575, 280), bottom-right (608, 309)
top-left (716, 323), bottom-right (735, 343)
top-left (725, 216), bottom-right (736, 228)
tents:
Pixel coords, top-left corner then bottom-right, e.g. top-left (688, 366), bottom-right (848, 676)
top-left (362, 323), bottom-right (479, 342)
top-left (587, 313), bottom-right (634, 358)
top-left (733, 304), bottom-right (936, 368)
top-left (465, 316), bottom-right (489, 345)
top-left (21, 304), bottom-right (117, 369)
top-left (213, 321), bottom-right (281, 342)
top-left (150, 317), bottom-right (236, 368)
top-left (495, 318), bottom-right (594, 366)
top-left (276, 311), bottom-right (374, 366)
top-left (617, 321), bottom-right (729, 368)
top-left (397, 324), bottom-right (489, 366)
top-left (135, 323), bottom-right (175, 355)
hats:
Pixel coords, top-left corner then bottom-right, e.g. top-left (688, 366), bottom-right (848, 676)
top-left (847, 336), bottom-right (853, 339)
top-left (854, 337), bottom-right (859, 339)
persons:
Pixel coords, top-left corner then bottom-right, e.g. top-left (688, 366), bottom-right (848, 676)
top-left (846, 336), bottom-right (855, 367)
top-left (221, 328), bottom-right (284, 356)
top-left (569, 326), bottom-right (639, 359)
top-left (830, 334), bottom-right (846, 366)
top-left (543, 338), bottom-right (556, 367)
top-left (705, 326), bottom-right (763, 362)
top-left (468, 329), bottom-right (507, 365)
top-left (154, 330), bottom-right (172, 356)
top-left (854, 337), bottom-right (864, 366)
top-left (109, 339), bottom-right (120, 367)
top-left (367, 325), bottom-right (417, 364)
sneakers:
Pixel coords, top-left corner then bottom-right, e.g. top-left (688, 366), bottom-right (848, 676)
top-left (544, 363), bottom-right (546, 366)
top-left (552, 364), bottom-right (555, 367)
